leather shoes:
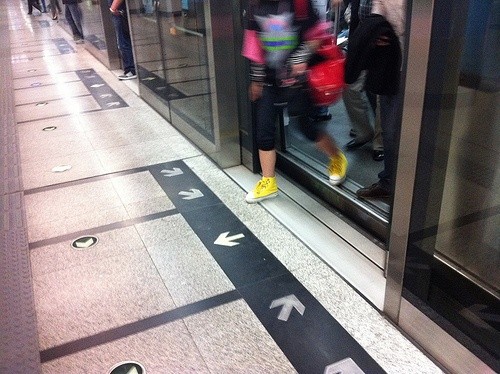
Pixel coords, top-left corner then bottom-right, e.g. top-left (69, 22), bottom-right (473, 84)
top-left (373, 150), bottom-right (384, 161)
top-left (348, 136), bottom-right (373, 149)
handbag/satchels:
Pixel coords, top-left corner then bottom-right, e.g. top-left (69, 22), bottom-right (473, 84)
top-left (307, 58), bottom-right (346, 105)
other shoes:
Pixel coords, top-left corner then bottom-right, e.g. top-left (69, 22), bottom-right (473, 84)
top-left (350, 129), bottom-right (358, 137)
top-left (313, 113), bottom-right (332, 122)
top-left (53, 16), bottom-right (57, 20)
top-left (75, 39), bottom-right (84, 44)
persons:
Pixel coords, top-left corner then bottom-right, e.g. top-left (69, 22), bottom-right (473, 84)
top-left (107, 0), bottom-right (137, 80)
top-left (181, 0), bottom-right (206, 38)
top-left (62, 0), bottom-right (84, 44)
top-left (282, 0), bottom-right (406, 196)
top-left (241, 0), bottom-right (349, 204)
top-left (28, 0), bottom-right (62, 20)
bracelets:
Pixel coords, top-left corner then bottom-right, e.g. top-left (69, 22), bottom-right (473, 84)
top-left (110, 8), bottom-right (116, 13)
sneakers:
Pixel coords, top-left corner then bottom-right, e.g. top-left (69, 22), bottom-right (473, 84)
top-left (118, 71), bottom-right (137, 80)
top-left (357, 181), bottom-right (393, 198)
top-left (245, 176), bottom-right (278, 203)
top-left (328, 152), bottom-right (348, 186)
